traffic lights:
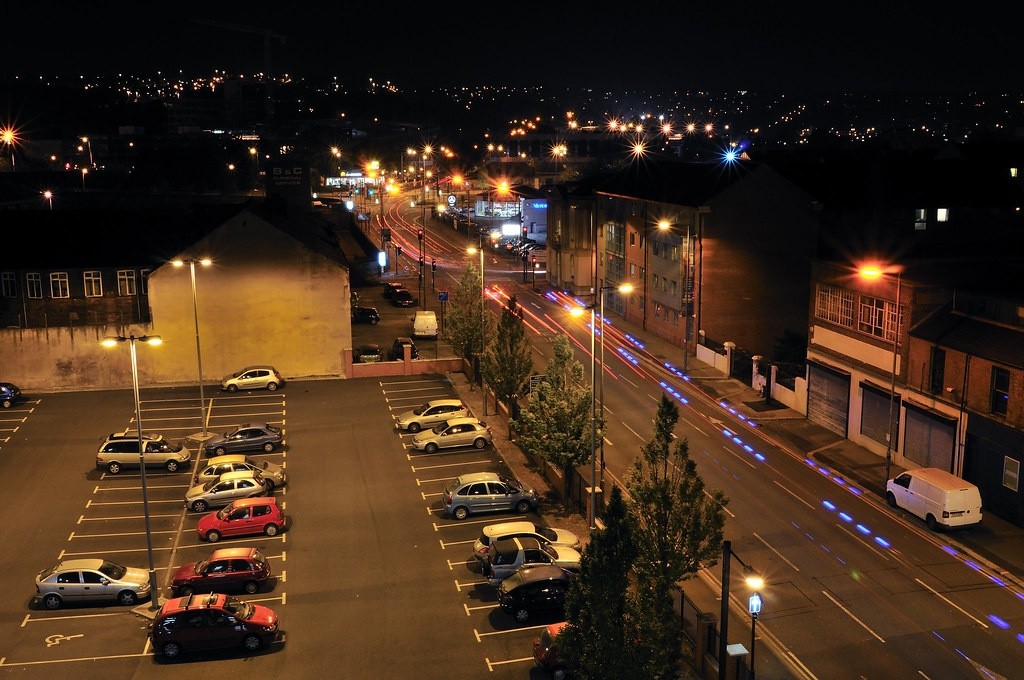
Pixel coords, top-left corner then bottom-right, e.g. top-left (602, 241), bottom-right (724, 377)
top-left (420, 256), bottom-right (424, 267)
top-left (397, 247), bottom-right (403, 254)
top-left (532, 258), bottom-right (537, 267)
top-left (523, 227), bottom-right (528, 237)
top-left (418, 229), bottom-right (422, 242)
top-left (432, 259), bottom-right (437, 272)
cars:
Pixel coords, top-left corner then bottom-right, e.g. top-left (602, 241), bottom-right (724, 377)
top-left (531, 619), bottom-right (642, 668)
top-left (145, 591), bottom-right (280, 660)
top-left (356, 344), bottom-right (383, 363)
top-left (205, 420), bottom-right (284, 456)
top-left (170, 546), bottom-right (273, 598)
top-left (352, 307), bottom-right (381, 325)
top-left (431, 205), bottom-right (548, 264)
top-left (384, 281), bottom-right (413, 306)
top-left (441, 471), bottom-right (540, 520)
top-left (216, 366), bottom-right (283, 392)
top-left (397, 398), bottom-right (470, 433)
top-left (0, 382), bottom-right (22, 409)
top-left (183, 453), bottom-right (287, 513)
top-left (34, 558), bottom-right (151, 609)
top-left (411, 416), bottom-right (492, 454)
top-left (197, 497), bottom-right (285, 543)
top-left (474, 521), bottom-right (590, 625)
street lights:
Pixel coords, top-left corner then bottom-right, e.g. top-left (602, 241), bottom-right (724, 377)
top-left (552, 147), bottom-right (561, 190)
top-left (168, 257), bottom-right (215, 438)
top-left (99, 331), bottom-right (172, 618)
top-left (719, 538), bottom-right (764, 680)
top-left (571, 307), bottom-right (598, 540)
top-left (656, 218), bottom-right (689, 370)
top-left (598, 282), bottom-right (634, 516)
top-left (358, 146), bottom-right (454, 262)
top-left (454, 177), bottom-right (471, 243)
top-left (862, 264), bottom-right (902, 496)
top-left (496, 186), bottom-right (520, 263)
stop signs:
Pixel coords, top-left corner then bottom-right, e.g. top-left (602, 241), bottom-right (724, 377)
top-left (507, 244), bottom-right (512, 250)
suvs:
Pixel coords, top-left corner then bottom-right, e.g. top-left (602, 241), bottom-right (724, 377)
top-left (392, 336), bottom-right (421, 362)
top-left (96, 427), bottom-right (192, 473)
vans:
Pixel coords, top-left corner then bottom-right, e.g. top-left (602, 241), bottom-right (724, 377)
top-left (411, 310), bottom-right (440, 342)
top-left (886, 467), bottom-right (986, 532)
